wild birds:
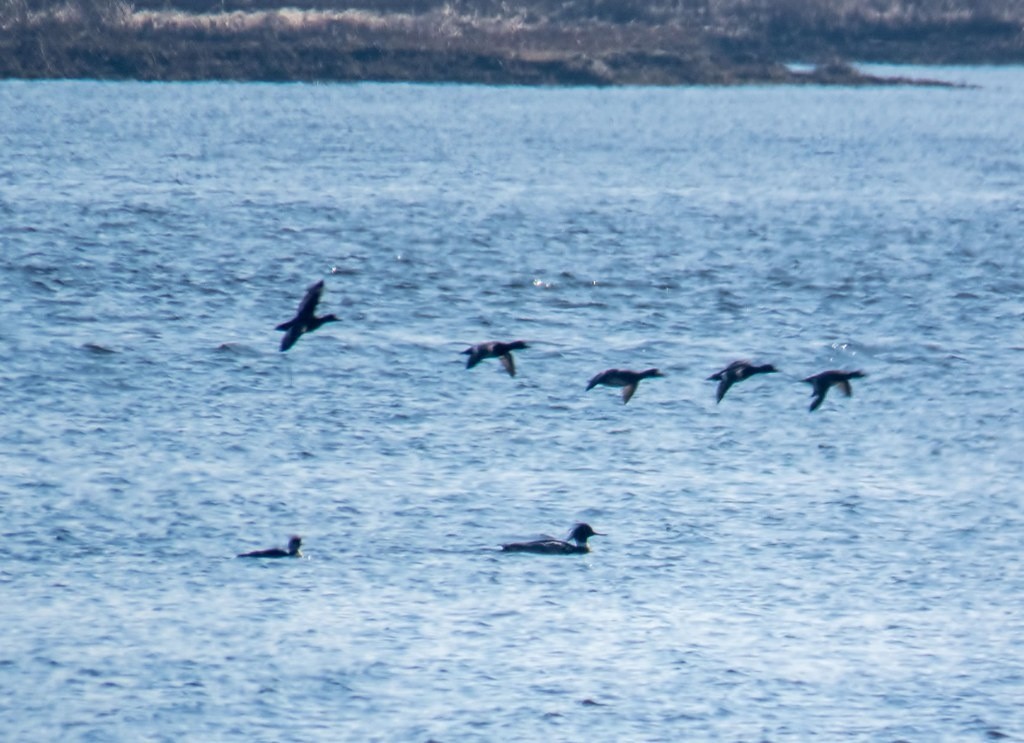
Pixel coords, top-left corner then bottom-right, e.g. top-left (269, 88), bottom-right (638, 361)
top-left (232, 535), bottom-right (305, 560)
top-left (457, 340), bottom-right (533, 378)
top-left (272, 278), bottom-right (343, 352)
top-left (585, 367), bottom-right (665, 405)
top-left (704, 360), bottom-right (783, 405)
top-left (795, 368), bottom-right (866, 413)
top-left (495, 522), bottom-right (609, 555)
